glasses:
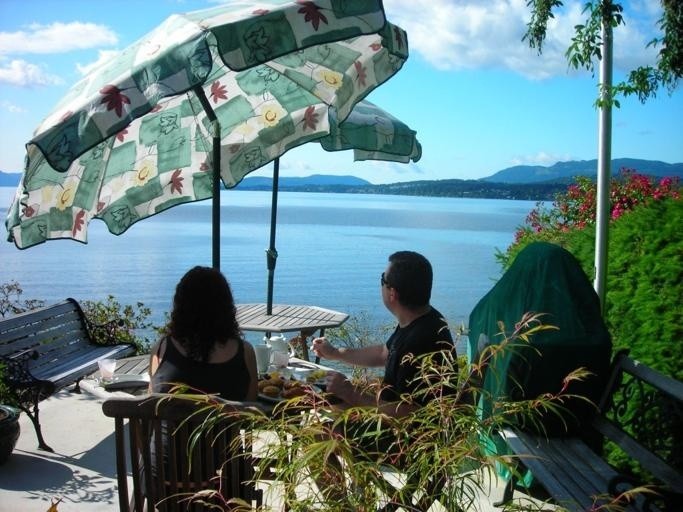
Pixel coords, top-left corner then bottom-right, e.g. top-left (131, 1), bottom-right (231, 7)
top-left (379, 272), bottom-right (390, 288)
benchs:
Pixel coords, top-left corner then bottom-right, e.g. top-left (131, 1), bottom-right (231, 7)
top-left (489, 346), bottom-right (682, 511)
top-left (0, 293), bottom-right (139, 452)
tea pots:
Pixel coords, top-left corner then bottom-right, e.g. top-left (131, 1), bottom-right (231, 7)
top-left (262, 335), bottom-right (295, 368)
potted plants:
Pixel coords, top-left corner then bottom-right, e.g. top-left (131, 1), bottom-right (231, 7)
top-left (0, 356), bottom-right (26, 467)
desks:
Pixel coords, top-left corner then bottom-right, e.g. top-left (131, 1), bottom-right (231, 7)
top-left (78, 350), bottom-right (343, 512)
top-left (228, 300), bottom-right (352, 367)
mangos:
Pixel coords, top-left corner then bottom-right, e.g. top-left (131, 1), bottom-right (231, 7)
top-left (263, 385), bottom-right (279, 396)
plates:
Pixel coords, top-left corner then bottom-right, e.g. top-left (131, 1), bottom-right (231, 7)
top-left (254, 379), bottom-right (322, 403)
top-left (286, 360), bottom-right (319, 373)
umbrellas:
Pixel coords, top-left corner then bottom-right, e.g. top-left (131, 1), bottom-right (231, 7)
top-left (265, 99), bottom-right (422, 338)
top-left (5, 0), bottom-right (408, 274)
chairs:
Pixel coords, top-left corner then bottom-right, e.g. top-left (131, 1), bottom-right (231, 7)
top-left (97, 393), bottom-right (270, 512)
top-left (345, 333), bottom-right (493, 511)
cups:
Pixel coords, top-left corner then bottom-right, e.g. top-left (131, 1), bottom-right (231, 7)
top-left (97, 359), bottom-right (116, 384)
top-left (254, 344), bottom-right (276, 374)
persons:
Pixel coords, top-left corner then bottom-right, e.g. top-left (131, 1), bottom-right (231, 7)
top-left (136, 268), bottom-right (258, 509)
top-left (302, 252), bottom-right (458, 512)
